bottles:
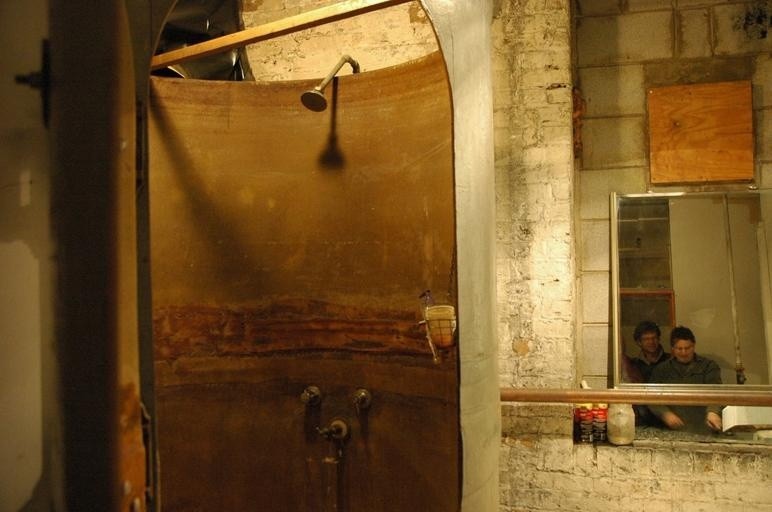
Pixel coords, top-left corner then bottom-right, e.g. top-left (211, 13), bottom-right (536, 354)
top-left (574, 402), bottom-right (607, 444)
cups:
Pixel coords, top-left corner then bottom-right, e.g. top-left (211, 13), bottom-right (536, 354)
top-left (425, 304), bottom-right (456, 347)
top-left (607, 402), bottom-right (637, 448)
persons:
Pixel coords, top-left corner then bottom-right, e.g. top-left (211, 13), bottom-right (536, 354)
top-left (645, 326), bottom-right (723, 435)
top-left (631, 320), bottom-right (672, 383)
